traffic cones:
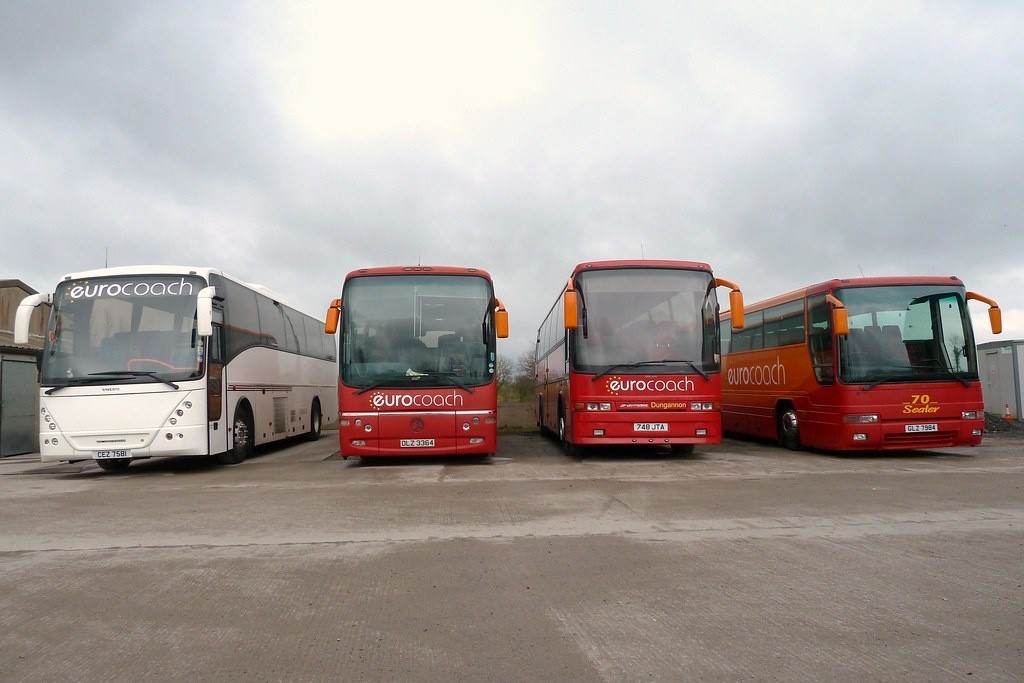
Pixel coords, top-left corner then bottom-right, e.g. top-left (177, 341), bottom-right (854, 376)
top-left (1004, 403), bottom-right (1014, 422)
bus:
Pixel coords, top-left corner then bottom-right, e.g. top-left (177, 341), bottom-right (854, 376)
top-left (325, 266), bottom-right (509, 458)
top-left (14, 265), bottom-right (338, 471)
top-left (681, 276), bottom-right (1002, 452)
top-left (209, 323), bottom-right (279, 422)
top-left (534, 259), bottom-right (744, 456)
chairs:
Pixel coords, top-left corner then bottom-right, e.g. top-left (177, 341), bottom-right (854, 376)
top-left (722, 323), bottom-right (910, 367)
